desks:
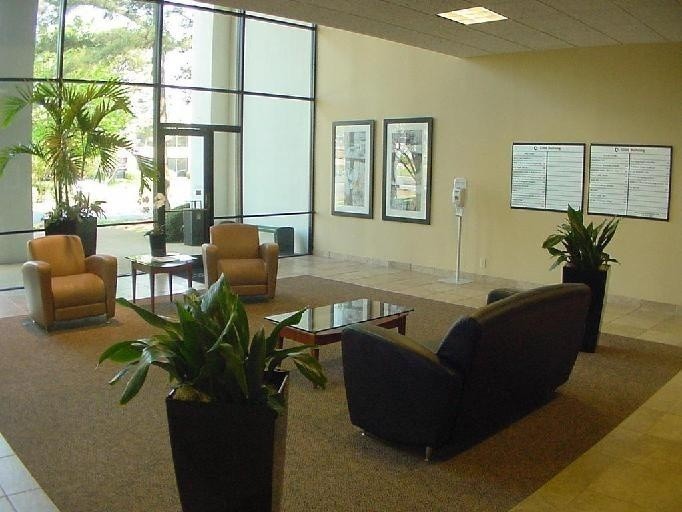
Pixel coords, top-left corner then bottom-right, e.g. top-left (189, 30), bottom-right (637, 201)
top-left (125, 253), bottom-right (198, 314)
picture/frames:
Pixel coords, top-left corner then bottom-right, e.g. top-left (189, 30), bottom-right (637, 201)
top-left (331, 116), bottom-right (433, 225)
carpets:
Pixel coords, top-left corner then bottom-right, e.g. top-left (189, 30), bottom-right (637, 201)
top-left (0, 272), bottom-right (682, 511)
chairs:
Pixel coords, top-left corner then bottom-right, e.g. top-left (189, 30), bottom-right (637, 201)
top-left (201, 221), bottom-right (280, 303)
top-left (20, 234), bottom-right (119, 334)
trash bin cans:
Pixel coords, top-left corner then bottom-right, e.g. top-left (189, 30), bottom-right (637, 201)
top-left (165, 200), bottom-right (207, 246)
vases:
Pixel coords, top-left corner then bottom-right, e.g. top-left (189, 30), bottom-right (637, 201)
top-left (149, 233), bottom-right (166, 257)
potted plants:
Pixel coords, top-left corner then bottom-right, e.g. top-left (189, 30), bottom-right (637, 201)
top-left (541, 201), bottom-right (621, 354)
top-left (0, 75), bottom-right (160, 257)
top-left (94, 271), bottom-right (330, 512)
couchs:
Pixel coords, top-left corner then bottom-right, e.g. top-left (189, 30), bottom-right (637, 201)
top-left (338, 269), bottom-right (591, 466)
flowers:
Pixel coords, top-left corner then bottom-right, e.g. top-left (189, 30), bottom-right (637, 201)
top-left (136, 192), bottom-right (171, 234)
